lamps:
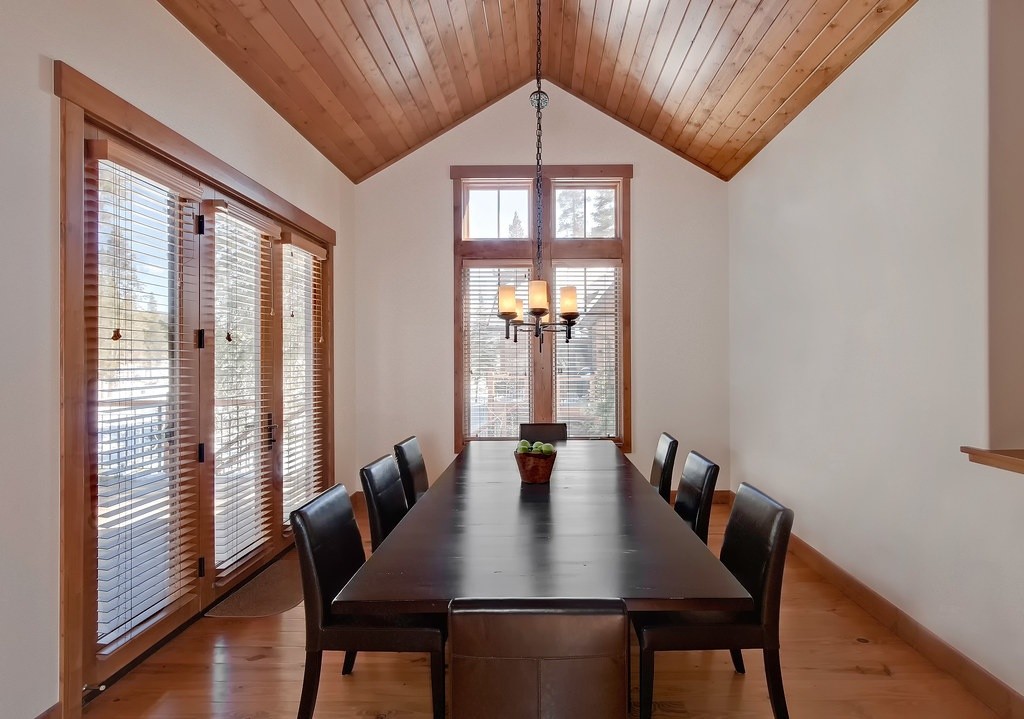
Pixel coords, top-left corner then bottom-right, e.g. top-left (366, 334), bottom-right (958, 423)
top-left (496, 0), bottom-right (580, 353)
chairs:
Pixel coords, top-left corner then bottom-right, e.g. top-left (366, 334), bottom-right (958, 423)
top-left (394, 436), bottom-right (430, 510)
top-left (633, 482), bottom-right (795, 719)
top-left (650, 432), bottom-right (678, 505)
top-left (519, 423), bottom-right (567, 441)
top-left (360, 454), bottom-right (409, 554)
top-left (445, 595), bottom-right (632, 719)
top-left (674, 450), bottom-right (719, 546)
top-left (289, 483), bottom-right (446, 719)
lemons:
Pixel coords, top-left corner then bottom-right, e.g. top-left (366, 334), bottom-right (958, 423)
top-left (516, 439), bottom-right (555, 455)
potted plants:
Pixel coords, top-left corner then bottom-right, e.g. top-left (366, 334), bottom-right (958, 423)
top-left (514, 439), bottom-right (557, 485)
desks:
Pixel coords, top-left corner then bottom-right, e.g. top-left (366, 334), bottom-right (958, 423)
top-left (330, 440), bottom-right (755, 611)
